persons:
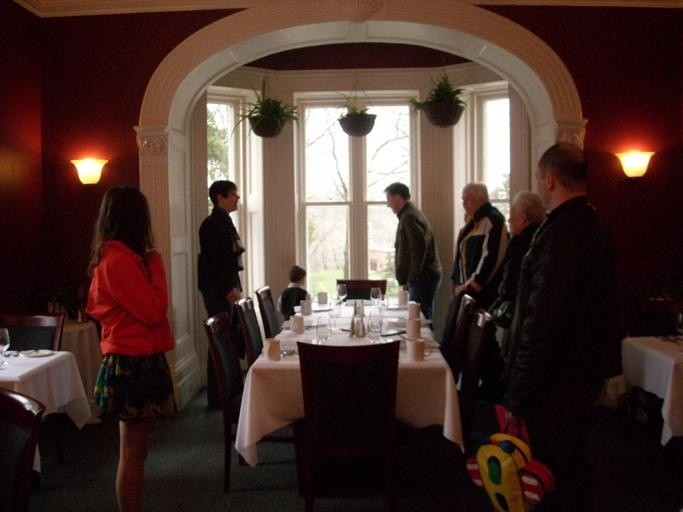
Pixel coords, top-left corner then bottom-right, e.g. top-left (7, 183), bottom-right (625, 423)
top-left (451, 142), bottom-right (622, 511)
top-left (276, 266), bottom-right (311, 321)
top-left (84, 186), bottom-right (177, 511)
top-left (384, 182), bottom-right (442, 332)
top-left (196, 181), bottom-right (245, 411)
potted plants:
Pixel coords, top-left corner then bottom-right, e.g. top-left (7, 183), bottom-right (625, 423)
top-left (410, 69), bottom-right (470, 128)
top-left (230, 75), bottom-right (302, 142)
top-left (335, 88), bottom-right (379, 137)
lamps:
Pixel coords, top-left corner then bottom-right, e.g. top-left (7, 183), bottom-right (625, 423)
top-left (615, 152), bottom-right (654, 178)
top-left (70, 157), bottom-right (109, 187)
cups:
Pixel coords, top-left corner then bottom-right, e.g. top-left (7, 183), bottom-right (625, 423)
top-left (675, 311), bottom-right (682, 335)
top-left (263, 290), bottom-right (328, 360)
top-left (396, 289), bottom-right (436, 365)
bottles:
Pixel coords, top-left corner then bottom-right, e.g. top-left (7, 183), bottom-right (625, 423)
top-left (73, 284), bottom-right (87, 325)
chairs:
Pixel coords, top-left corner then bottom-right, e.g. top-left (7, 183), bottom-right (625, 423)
top-left (616, 295), bottom-right (683, 337)
top-left (0, 390), bottom-right (46, 511)
top-left (295, 336), bottom-right (397, 512)
top-left (0, 314), bottom-right (65, 353)
top-left (234, 296), bottom-right (263, 375)
top-left (204, 311), bottom-right (296, 489)
top-left (335, 280), bottom-right (384, 300)
top-left (441, 282), bottom-right (466, 351)
top-left (449, 311), bottom-right (492, 432)
top-left (440, 294), bottom-right (477, 363)
top-left (255, 284), bottom-right (280, 340)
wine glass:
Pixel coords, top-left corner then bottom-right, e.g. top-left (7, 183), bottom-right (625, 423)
top-left (316, 285), bottom-right (391, 341)
top-left (0, 327), bottom-right (12, 371)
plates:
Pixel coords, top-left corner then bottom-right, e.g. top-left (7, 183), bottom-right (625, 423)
top-left (22, 348), bottom-right (55, 358)
